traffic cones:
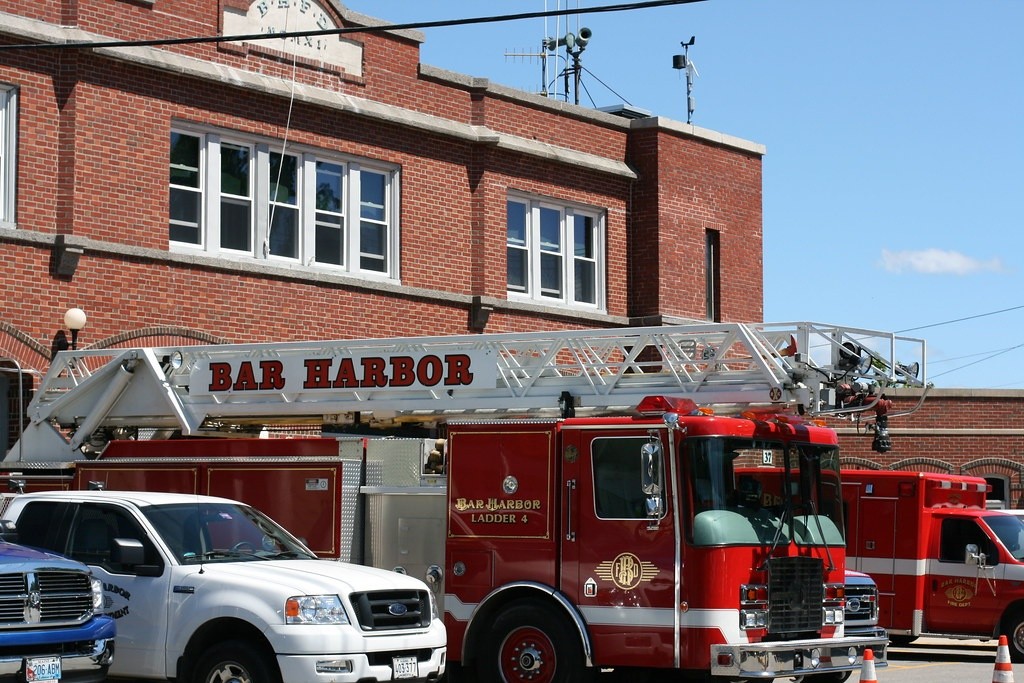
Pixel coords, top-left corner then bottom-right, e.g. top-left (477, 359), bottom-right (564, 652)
top-left (985, 628), bottom-right (1019, 681)
top-left (856, 644), bottom-right (884, 678)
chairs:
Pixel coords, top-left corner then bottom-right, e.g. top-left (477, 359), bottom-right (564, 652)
top-left (596, 461), bottom-right (644, 518)
top-left (71, 519), bottom-right (119, 568)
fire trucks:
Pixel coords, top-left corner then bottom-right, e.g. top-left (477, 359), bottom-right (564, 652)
top-left (832, 467), bottom-right (1023, 658)
top-left (0, 318), bottom-right (929, 682)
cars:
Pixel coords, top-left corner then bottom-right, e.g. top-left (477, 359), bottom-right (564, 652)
top-left (1, 535), bottom-right (118, 678)
top-left (0, 488), bottom-right (452, 682)
top-left (838, 557), bottom-right (888, 667)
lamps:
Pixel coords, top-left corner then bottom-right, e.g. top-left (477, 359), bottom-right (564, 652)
top-left (64, 308), bottom-right (87, 361)
